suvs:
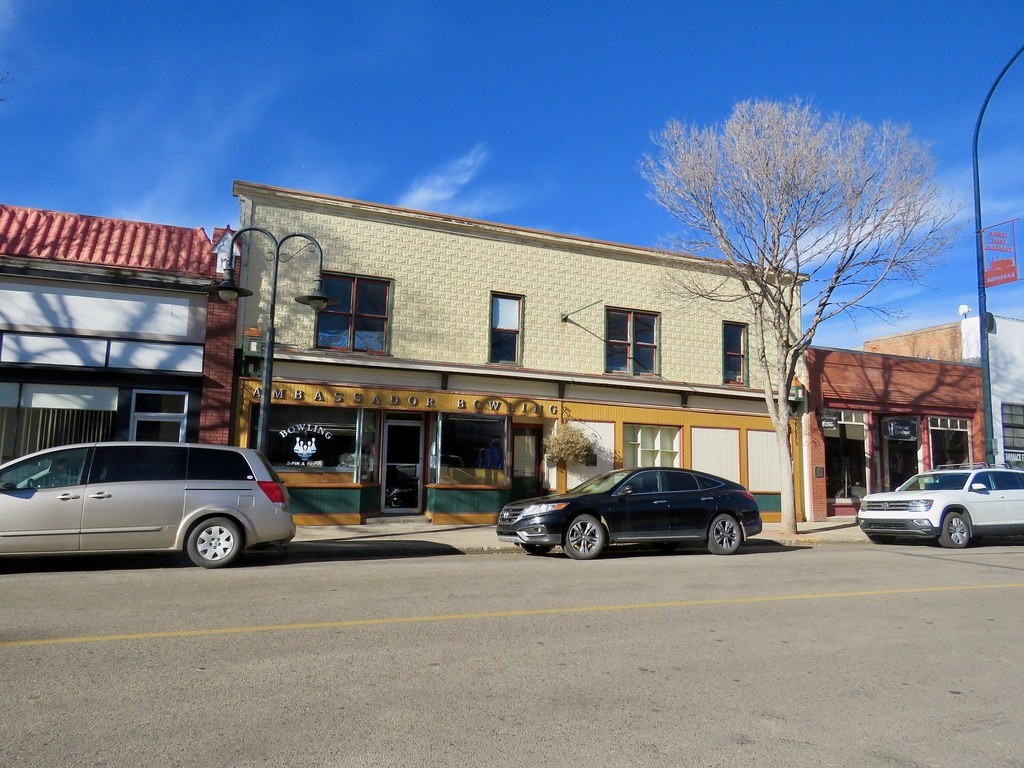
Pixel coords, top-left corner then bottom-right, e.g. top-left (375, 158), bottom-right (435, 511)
top-left (857, 462), bottom-right (1024, 550)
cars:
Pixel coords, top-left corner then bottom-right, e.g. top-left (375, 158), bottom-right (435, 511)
top-left (496, 466), bottom-right (762, 561)
top-left (0, 441), bottom-right (296, 571)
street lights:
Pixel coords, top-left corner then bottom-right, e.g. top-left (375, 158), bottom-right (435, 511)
top-left (212, 226), bottom-right (329, 458)
